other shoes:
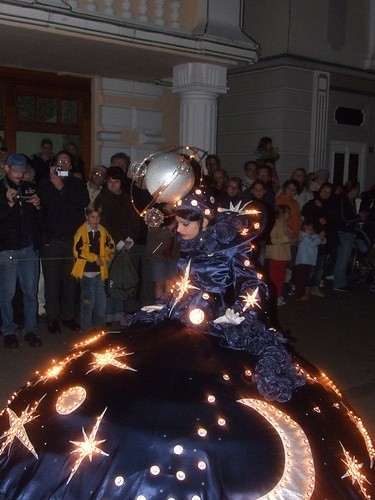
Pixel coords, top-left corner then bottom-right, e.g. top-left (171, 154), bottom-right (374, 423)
top-left (62, 319), bottom-right (81, 332)
top-left (48, 321), bottom-right (62, 334)
top-left (25, 332), bottom-right (43, 347)
top-left (310, 285), bottom-right (326, 298)
top-left (294, 293), bottom-right (310, 302)
top-left (277, 297), bottom-right (287, 306)
top-left (4, 334), bottom-right (18, 349)
top-left (334, 286), bottom-right (351, 293)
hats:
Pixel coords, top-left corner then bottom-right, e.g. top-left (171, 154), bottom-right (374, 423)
top-left (314, 170), bottom-right (330, 178)
top-left (5, 154), bottom-right (27, 168)
top-left (105, 166), bottom-right (122, 179)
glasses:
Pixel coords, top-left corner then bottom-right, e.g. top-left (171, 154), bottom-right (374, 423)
top-left (94, 172), bottom-right (103, 177)
top-left (58, 160), bottom-right (69, 164)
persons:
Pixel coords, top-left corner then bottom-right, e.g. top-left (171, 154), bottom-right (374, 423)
top-left (141, 184), bottom-right (270, 326)
top-left (0, 137), bottom-right (375, 350)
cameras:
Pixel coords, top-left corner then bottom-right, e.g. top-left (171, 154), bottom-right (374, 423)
top-left (12, 193), bottom-right (32, 201)
top-left (55, 167), bottom-right (69, 177)
top-left (115, 240), bottom-right (125, 251)
